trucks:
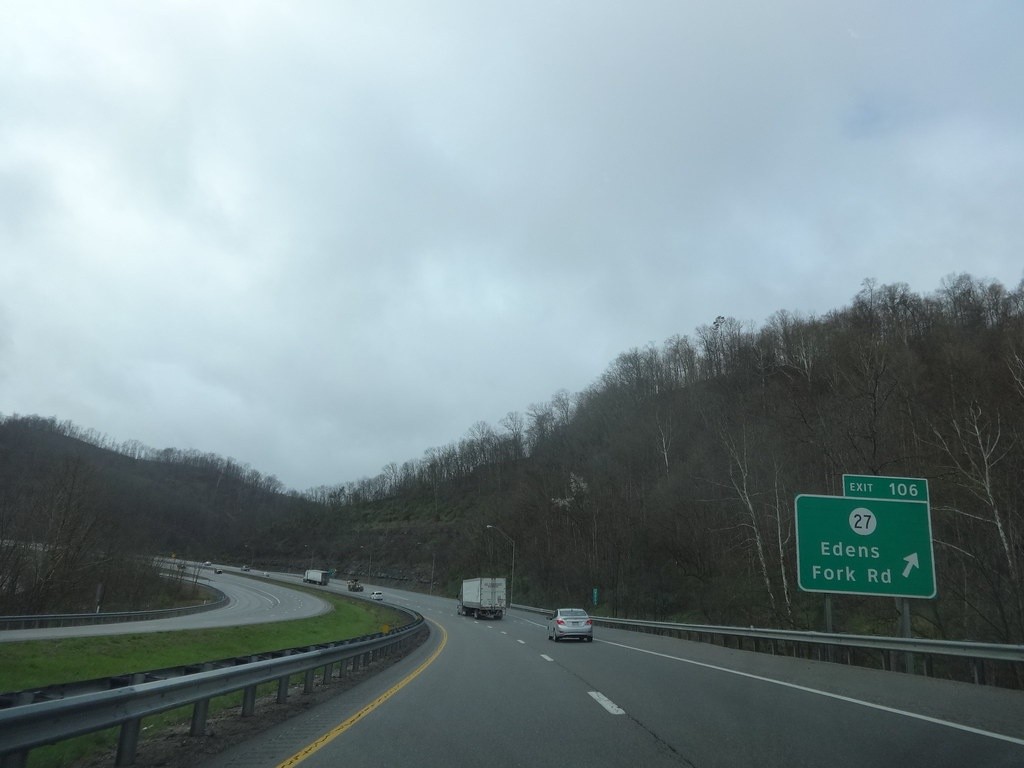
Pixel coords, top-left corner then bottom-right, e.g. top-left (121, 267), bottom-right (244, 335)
top-left (456, 577), bottom-right (507, 619)
top-left (303, 570), bottom-right (330, 586)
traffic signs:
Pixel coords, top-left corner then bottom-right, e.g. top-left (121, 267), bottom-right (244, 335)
top-left (794, 474), bottom-right (938, 600)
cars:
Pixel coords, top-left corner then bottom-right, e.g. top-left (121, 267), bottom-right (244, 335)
top-left (348, 581), bottom-right (363, 592)
top-left (205, 562), bottom-right (211, 566)
top-left (177, 564), bottom-right (187, 569)
top-left (261, 571), bottom-right (269, 577)
top-left (241, 566), bottom-right (250, 571)
top-left (545, 608), bottom-right (593, 643)
top-left (370, 591), bottom-right (383, 600)
top-left (215, 568), bottom-right (222, 573)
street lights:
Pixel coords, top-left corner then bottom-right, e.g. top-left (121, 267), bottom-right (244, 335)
top-left (305, 544), bottom-right (313, 570)
top-left (487, 524), bottom-right (516, 609)
top-left (361, 546), bottom-right (372, 584)
